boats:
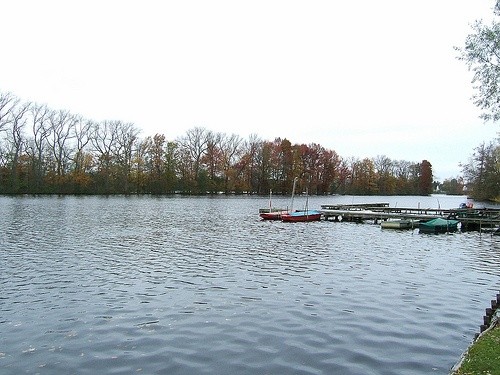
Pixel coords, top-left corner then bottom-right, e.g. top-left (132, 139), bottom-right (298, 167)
top-left (258, 207), bottom-right (322, 221)
top-left (381, 216), bottom-right (463, 233)
